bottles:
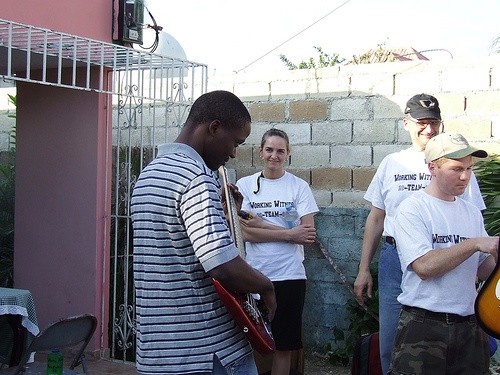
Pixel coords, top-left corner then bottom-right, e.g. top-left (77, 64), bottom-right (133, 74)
top-left (47, 346), bottom-right (64, 375)
top-left (285, 204), bottom-right (301, 229)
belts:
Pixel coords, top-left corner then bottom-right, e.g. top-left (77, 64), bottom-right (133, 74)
top-left (402, 305), bottom-right (470, 322)
top-left (386, 236), bottom-right (396, 244)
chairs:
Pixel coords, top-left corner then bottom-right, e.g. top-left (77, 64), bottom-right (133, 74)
top-left (0, 313), bottom-right (98, 375)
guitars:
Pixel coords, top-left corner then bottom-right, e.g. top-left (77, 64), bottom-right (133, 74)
top-left (473, 232), bottom-right (500, 340)
top-left (210, 165), bottom-right (277, 356)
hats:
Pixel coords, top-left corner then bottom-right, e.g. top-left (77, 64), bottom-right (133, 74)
top-left (405, 93), bottom-right (441, 120)
top-left (425, 133), bottom-right (488, 164)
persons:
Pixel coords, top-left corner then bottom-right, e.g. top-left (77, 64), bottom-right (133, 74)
top-left (131, 90), bottom-right (278, 375)
top-left (387, 133), bottom-right (500, 375)
top-left (353, 94), bottom-right (487, 375)
top-left (235, 128), bottom-right (320, 375)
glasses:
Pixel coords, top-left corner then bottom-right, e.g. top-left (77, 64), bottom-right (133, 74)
top-left (407, 118), bottom-right (443, 128)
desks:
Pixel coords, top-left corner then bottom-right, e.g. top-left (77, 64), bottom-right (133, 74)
top-left (0, 287), bottom-right (41, 372)
